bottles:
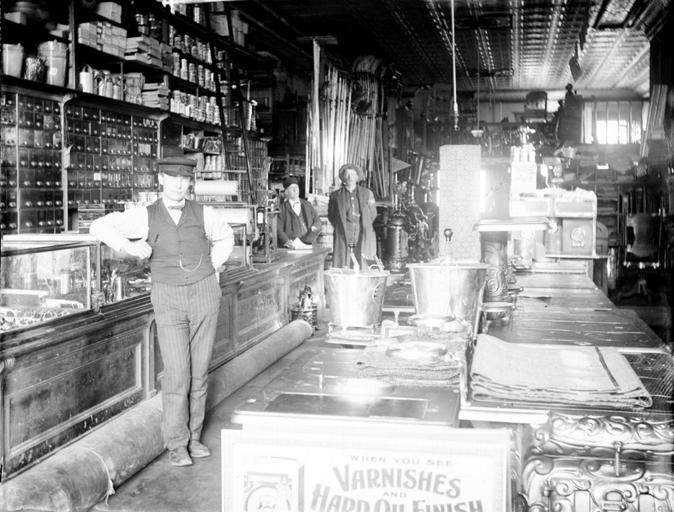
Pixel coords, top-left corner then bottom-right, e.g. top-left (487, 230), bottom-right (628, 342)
top-left (112, 271), bottom-right (125, 301)
top-left (437, 229), bottom-right (458, 265)
top-left (56, 268), bottom-right (72, 296)
top-left (76, 63), bottom-right (124, 101)
top-left (168, 24), bottom-right (222, 125)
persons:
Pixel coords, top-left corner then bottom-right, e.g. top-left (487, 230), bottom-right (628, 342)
top-left (276, 177), bottom-right (324, 249)
top-left (327, 164), bottom-right (378, 268)
top-left (89, 156), bottom-right (235, 467)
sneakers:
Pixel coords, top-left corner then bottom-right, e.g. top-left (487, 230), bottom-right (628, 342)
top-left (169, 447), bottom-right (192, 466)
top-left (188, 441), bottom-right (210, 457)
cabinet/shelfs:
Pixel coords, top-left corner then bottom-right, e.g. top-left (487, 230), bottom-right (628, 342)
top-left (509, 158), bottom-right (674, 298)
top-left (0, 1), bottom-right (333, 481)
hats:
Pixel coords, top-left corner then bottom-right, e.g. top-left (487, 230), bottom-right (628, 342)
top-left (157, 158), bottom-right (196, 177)
top-left (339, 164), bottom-right (362, 183)
top-left (283, 177), bottom-right (299, 188)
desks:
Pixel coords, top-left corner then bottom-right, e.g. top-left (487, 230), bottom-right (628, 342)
top-left (219, 274), bottom-right (674, 512)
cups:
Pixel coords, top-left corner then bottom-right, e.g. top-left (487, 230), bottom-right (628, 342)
top-left (38, 40), bottom-right (68, 86)
top-left (2, 42), bottom-right (25, 77)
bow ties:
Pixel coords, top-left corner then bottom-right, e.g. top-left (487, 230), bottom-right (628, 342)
top-left (169, 204), bottom-right (184, 211)
